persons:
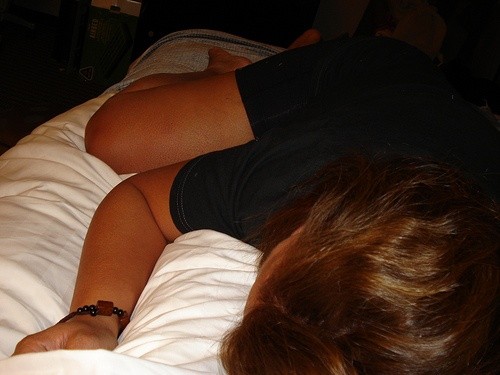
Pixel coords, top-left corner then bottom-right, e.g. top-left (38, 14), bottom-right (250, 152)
top-left (11, 26), bottom-right (500, 375)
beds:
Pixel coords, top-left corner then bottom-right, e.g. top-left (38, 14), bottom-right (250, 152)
top-left (1, 26), bottom-right (500, 375)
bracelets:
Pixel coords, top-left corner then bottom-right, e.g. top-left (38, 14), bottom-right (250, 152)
top-left (55, 300), bottom-right (131, 337)
